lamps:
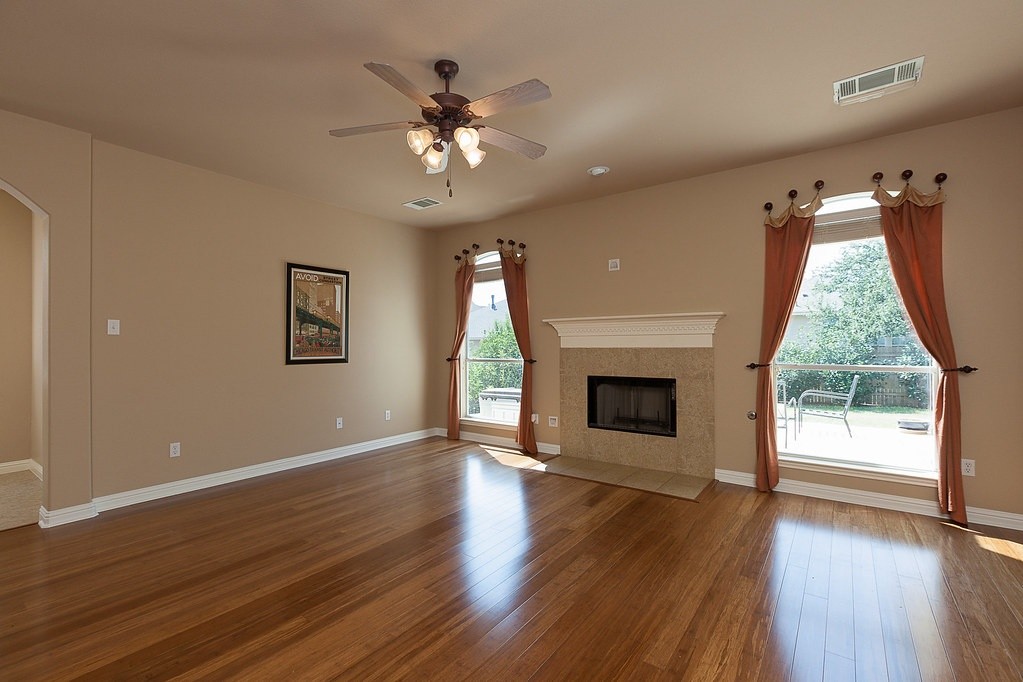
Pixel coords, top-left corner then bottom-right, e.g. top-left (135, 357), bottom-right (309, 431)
top-left (407, 127), bottom-right (487, 174)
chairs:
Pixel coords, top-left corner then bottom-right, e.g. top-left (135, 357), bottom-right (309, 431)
top-left (777, 381), bottom-right (797, 449)
top-left (797, 374), bottom-right (860, 438)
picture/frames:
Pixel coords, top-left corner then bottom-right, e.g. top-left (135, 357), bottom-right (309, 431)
top-left (286, 263), bottom-right (349, 364)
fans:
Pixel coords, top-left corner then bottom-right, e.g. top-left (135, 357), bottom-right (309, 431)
top-left (329, 59), bottom-right (552, 160)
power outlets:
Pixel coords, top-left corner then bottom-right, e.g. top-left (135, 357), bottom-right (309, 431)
top-left (961, 459), bottom-right (975, 476)
top-left (170, 442), bottom-right (180, 458)
